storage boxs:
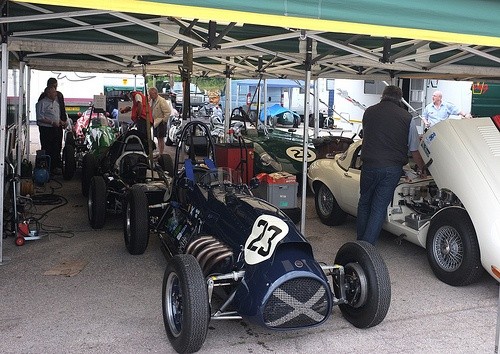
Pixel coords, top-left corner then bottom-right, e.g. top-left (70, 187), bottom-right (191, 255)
top-left (250, 178), bottom-right (299, 209)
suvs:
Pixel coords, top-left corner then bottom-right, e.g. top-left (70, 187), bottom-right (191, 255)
top-left (155, 79), bottom-right (209, 114)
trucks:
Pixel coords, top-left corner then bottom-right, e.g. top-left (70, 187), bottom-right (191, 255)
top-left (217, 79), bottom-right (285, 116)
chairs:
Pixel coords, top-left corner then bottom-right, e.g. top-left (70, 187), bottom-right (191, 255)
top-left (176, 168), bottom-right (216, 208)
top-left (120, 153), bottom-right (148, 187)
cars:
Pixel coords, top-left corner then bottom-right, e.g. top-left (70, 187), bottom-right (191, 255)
top-left (307, 117), bottom-right (500, 287)
top-left (63, 103), bottom-right (391, 354)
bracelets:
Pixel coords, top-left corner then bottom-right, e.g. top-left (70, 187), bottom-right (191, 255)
top-left (51, 121), bottom-right (54, 125)
top-left (463, 113), bottom-right (467, 117)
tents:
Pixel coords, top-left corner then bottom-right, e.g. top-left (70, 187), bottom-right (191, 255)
top-left (1, 0), bottom-right (500, 262)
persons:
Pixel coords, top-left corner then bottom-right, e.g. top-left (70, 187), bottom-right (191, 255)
top-left (421, 91), bottom-right (473, 132)
top-left (130, 87), bottom-right (170, 157)
top-left (355, 85), bottom-right (427, 246)
top-left (34, 76), bottom-right (69, 170)
